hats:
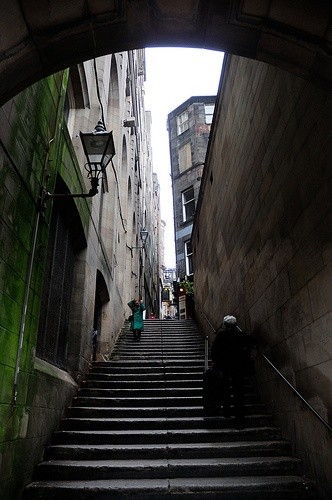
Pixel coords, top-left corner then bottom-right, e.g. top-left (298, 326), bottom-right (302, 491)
top-left (223, 315), bottom-right (237, 323)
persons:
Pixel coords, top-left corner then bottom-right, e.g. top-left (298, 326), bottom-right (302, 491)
top-left (212, 315), bottom-right (246, 415)
top-left (127, 298), bottom-right (146, 340)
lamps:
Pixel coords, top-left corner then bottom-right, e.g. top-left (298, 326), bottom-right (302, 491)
top-left (45, 119), bottom-right (116, 199)
top-left (131, 227), bottom-right (149, 248)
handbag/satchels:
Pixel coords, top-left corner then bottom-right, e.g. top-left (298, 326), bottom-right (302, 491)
top-left (128, 315), bottom-right (134, 321)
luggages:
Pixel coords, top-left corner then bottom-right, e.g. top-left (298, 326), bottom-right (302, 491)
top-left (203, 367), bottom-right (221, 415)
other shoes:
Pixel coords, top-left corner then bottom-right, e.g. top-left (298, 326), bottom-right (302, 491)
top-left (135, 339), bottom-right (139, 341)
top-left (223, 409), bottom-right (230, 417)
top-left (237, 417), bottom-right (245, 426)
